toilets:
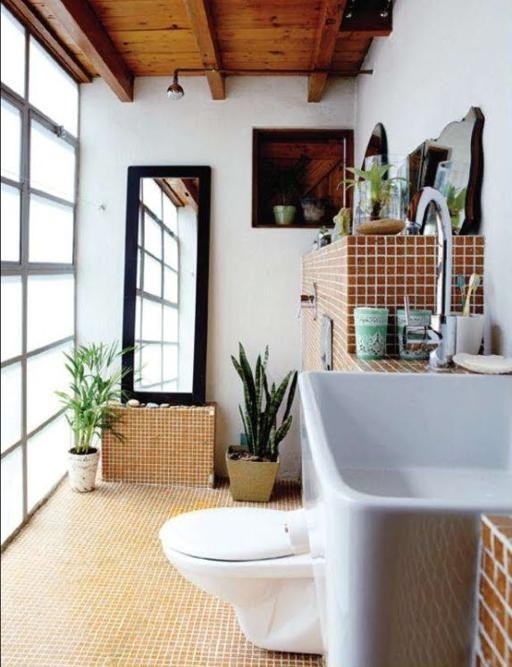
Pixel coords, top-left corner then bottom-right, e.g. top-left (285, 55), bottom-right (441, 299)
top-left (159, 505), bottom-right (321, 655)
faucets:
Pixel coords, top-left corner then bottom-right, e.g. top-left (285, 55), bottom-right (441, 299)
top-left (404, 187), bottom-right (453, 366)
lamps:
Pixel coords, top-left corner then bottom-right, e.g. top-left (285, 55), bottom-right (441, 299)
top-left (168, 67), bottom-right (373, 100)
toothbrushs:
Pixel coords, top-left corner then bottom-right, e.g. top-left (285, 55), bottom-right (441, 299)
top-left (462, 274), bottom-right (481, 316)
top-left (458, 273), bottom-right (466, 306)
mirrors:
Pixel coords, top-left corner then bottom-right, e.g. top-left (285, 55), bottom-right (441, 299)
top-left (361, 124), bottom-right (390, 183)
top-left (120, 165), bottom-right (212, 406)
top-left (424, 106), bottom-right (483, 234)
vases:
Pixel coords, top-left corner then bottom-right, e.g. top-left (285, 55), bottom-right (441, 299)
top-left (303, 198), bottom-right (326, 223)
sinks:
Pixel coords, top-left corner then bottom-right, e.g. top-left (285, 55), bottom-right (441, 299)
top-left (299, 371), bottom-right (510, 666)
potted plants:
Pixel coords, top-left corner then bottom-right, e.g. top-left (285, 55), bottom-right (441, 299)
top-left (265, 164), bottom-right (295, 226)
top-left (55, 341), bottom-right (118, 493)
top-left (226, 341), bottom-right (297, 501)
top-left (339, 164), bottom-right (402, 234)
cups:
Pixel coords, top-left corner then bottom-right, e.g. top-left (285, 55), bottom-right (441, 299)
top-left (442, 311), bottom-right (485, 356)
top-left (395, 310), bottom-right (431, 360)
top-left (354, 308), bottom-right (389, 360)
